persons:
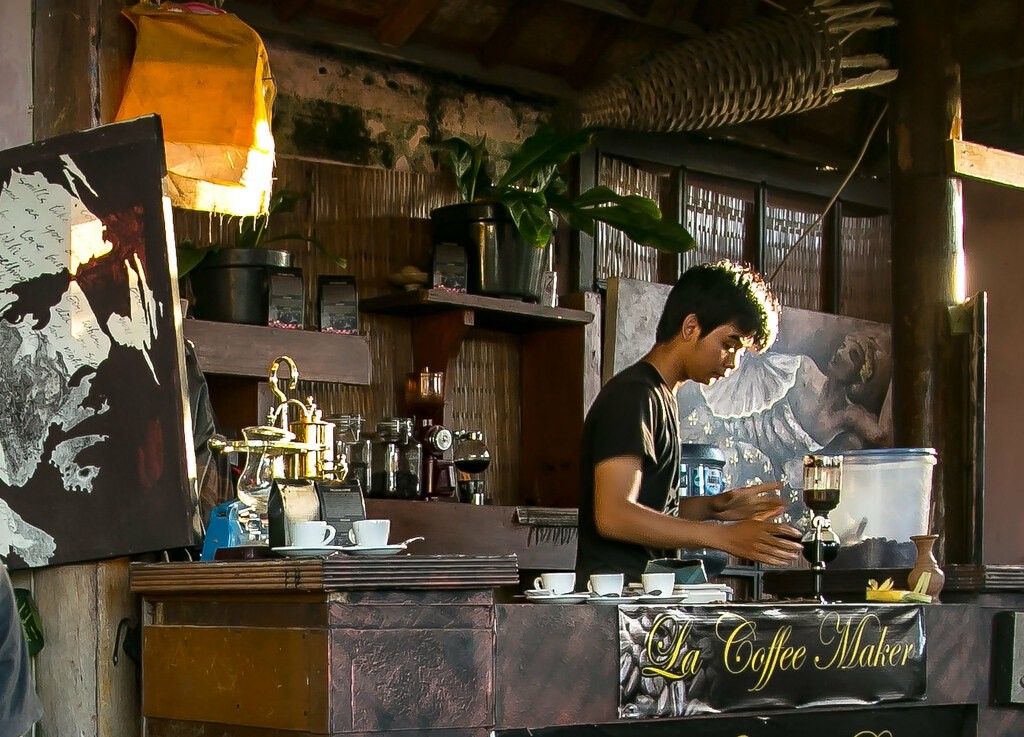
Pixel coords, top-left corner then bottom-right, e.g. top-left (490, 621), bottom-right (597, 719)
top-left (1, 557), bottom-right (44, 736)
top-left (574, 257), bottom-right (804, 593)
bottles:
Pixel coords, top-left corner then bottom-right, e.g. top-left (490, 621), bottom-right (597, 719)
top-left (908, 534), bottom-right (945, 605)
top-left (680, 445), bottom-right (728, 578)
top-left (336, 416), bottom-right (423, 500)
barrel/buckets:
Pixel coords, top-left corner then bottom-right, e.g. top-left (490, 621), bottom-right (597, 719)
top-left (826, 448), bottom-right (937, 566)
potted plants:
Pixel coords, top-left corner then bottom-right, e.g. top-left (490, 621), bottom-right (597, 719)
top-left (176, 175), bottom-right (333, 323)
top-left (421, 110), bottom-right (698, 299)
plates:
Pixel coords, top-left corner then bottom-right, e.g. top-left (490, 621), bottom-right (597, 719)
top-left (340, 547), bottom-right (404, 554)
top-left (527, 595), bottom-right (590, 603)
top-left (270, 546), bottom-right (341, 558)
top-left (633, 596), bottom-right (687, 603)
top-left (585, 598), bottom-right (639, 604)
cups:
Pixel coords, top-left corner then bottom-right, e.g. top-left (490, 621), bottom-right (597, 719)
top-left (353, 520), bottom-right (390, 547)
top-left (642, 573), bottom-right (675, 597)
top-left (293, 521), bottom-right (335, 545)
top-left (534, 573), bottom-right (576, 595)
top-left (587, 574), bottom-right (624, 597)
